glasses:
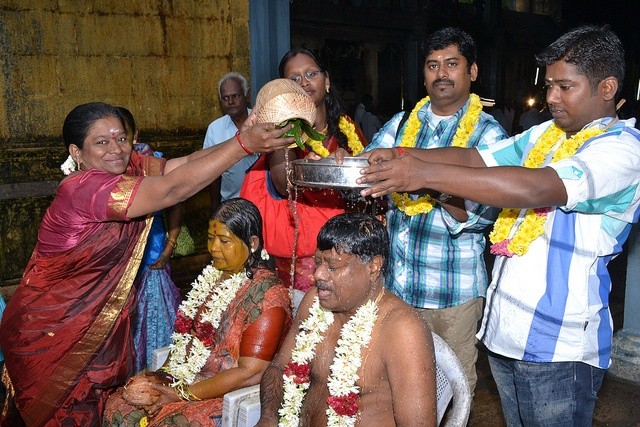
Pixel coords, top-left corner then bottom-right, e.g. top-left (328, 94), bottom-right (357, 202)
top-left (291, 71), bottom-right (324, 83)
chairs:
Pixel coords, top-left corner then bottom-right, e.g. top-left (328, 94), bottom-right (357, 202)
top-left (153, 290), bottom-right (472, 427)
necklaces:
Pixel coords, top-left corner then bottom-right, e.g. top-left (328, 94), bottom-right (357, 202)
top-left (292, 114), bottom-right (365, 159)
top-left (485, 114), bottom-right (620, 260)
top-left (390, 93), bottom-right (484, 217)
top-left (167, 260), bottom-right (252, 386)
top-left (277, 285), bottom-right (386, 427)
top-left (309, 122), bottom-right (329, 135)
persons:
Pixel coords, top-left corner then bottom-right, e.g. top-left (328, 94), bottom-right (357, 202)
top-left (315, 81), bottom-right (640, 315)
top-left (238, 46), bottom-right (370, 325)
top-left (327, 26), bottom-right (513, 427)
top-left (255, 210), bottom-right (438, 426)
top-left (356, 23), bottom-right (640, 426)
top-left (109, 106), bottom-right (185, 388)
top-left (101, 196), bottom-right (293, 427)
top-left (201, 71), bottom-right (265, 204)
top-left (0, 101), bottom-right (298, 427)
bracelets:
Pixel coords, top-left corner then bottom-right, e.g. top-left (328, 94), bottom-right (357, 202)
top-left (286, 163), bottom-right (305, 191)
top-left (155, 366), bottom-right (170, 374)
top-left (234, 132), bottom-right (256, 155)
top-left (174, 382), bottom-right (202, 402)
top-left (395, 146), bottom-right (407, 157)
top-left (166, 236), bottom-right (177, 249)
